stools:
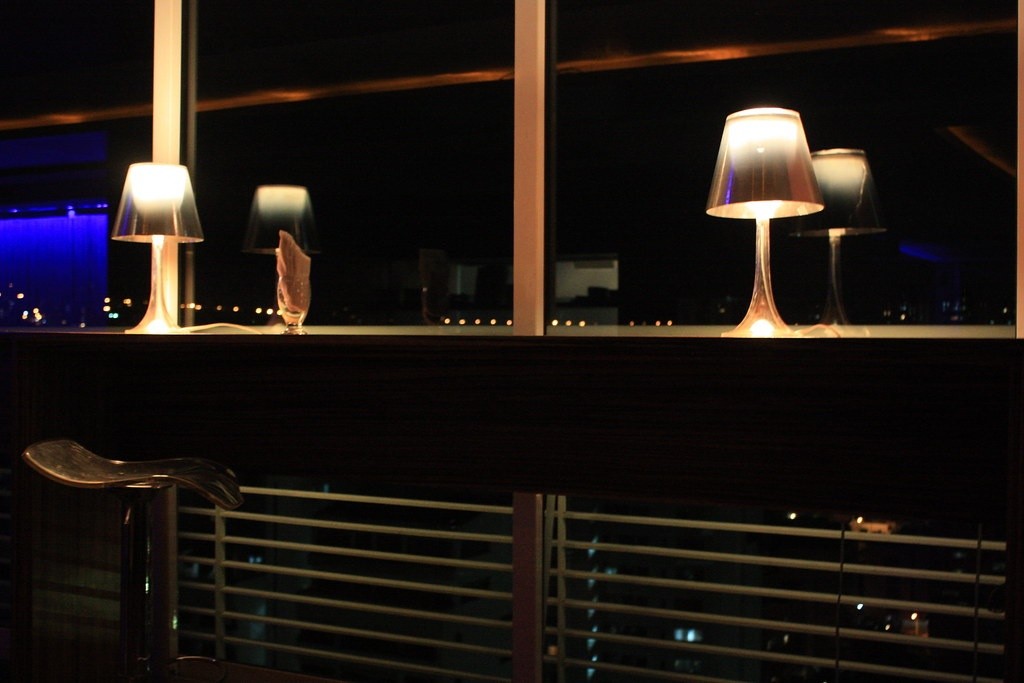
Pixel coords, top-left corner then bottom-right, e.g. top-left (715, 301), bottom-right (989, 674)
top-left (21, 440), bottom-right (243, 683)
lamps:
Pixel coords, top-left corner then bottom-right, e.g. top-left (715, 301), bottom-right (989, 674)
top-left (241, 184), bottom-right (320, 255)
top-left (705, 107), bottom-right (826, 336)
top-left (789, 148), bottom-right (888, 325)
top-left (112, 162), bottom-right (205, 333)
